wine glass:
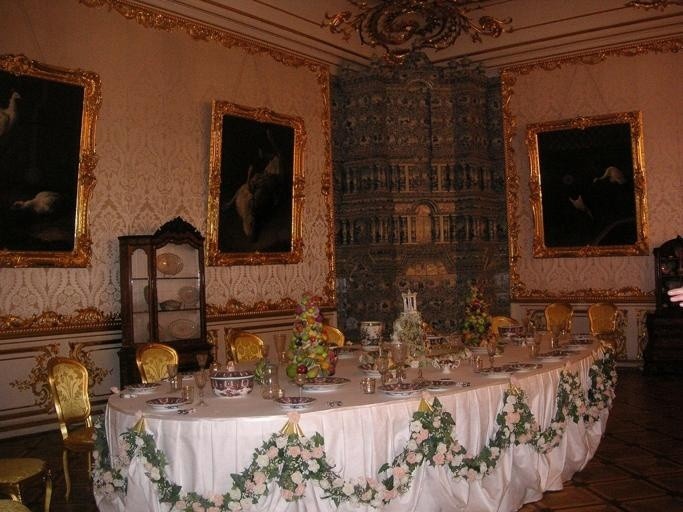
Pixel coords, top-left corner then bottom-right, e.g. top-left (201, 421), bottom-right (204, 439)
top-left (295, 374), bottom-right (306, 397)
top-left (392, 344), bottom-right (408, 379)
top-left (487, 344), bottom-right (496, 367)
top-left (167, 353), bottom-right (207, 407)
top-left (259, 335), bottom-right (286, 370)
top-left (377, 359), bottom-right (389, 384)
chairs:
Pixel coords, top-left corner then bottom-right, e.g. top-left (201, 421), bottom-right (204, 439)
top-left (47, 357), bottom-right (100, 497)
top-left (2, 499), bottom-right (28, 511)
top-left (2, 456), bottom-right (53, 509)
top-left (586, 304), bottom-right (617, 334)
top-left (544, 304), bottom-right (573, 333)
top-left (324, 325), bottom-right (344, 347)
top-left (227, 331), bottom-right (265, 362)
top-left (135, 343), bottom-right (178, 384)
top-left (490, 317), bottom-right (518, 335)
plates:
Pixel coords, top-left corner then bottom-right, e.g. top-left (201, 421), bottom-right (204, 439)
top-left (145, 396), bottom-right (189, 410)
top-left (359, 364), bottom-right (410, 378)
top-left (378, 379), bottom-right (457, 397)
top-left (178, 286), bottom-right (199, 305)
top-left (168, 319), bottom-right (199, 340)
top-left (273, 395), bottom-right (316, 409)
top-left (479, 364), bottom-right (543, 378)
top-left (124, 382), bottom-right (162, 394)
top-left (303, 376), bottom-right (351, 392)
top-left (157, 253), bottom-right (184, 275)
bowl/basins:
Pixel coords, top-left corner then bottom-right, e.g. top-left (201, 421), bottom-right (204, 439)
top-left (209, 369), bottom-right (254, 399)
top-left (160, 299), bottom-right (182, 311)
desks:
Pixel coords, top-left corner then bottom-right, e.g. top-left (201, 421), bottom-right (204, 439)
top-left (91, 339), bottom-right (619, 511)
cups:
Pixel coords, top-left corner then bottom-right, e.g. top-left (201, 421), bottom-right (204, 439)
top-left (359, 377), bottom-right (376, 394)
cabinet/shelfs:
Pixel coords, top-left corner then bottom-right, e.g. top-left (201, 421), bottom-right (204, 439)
top-left (643, 236), bottom-right (683, 376)
top-left (118, 215), bottom-right (213, 388)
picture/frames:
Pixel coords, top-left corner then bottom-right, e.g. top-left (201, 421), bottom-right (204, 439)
top-left (525, 110), bottom-right (650, 258)
top-left (1, 54), bottom-right (101, 268)
top-left (206, 100), bottom-right (306, 266)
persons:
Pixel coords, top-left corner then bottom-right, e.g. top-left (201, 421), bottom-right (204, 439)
top-left (555, 181), bottom-right (594, 243)
top-left (667, 285), bottom-right (683, 307)
top-left (219, 149), bottom-right (288, 242)
top-left (595, 162), bottom-right (627, 188)
